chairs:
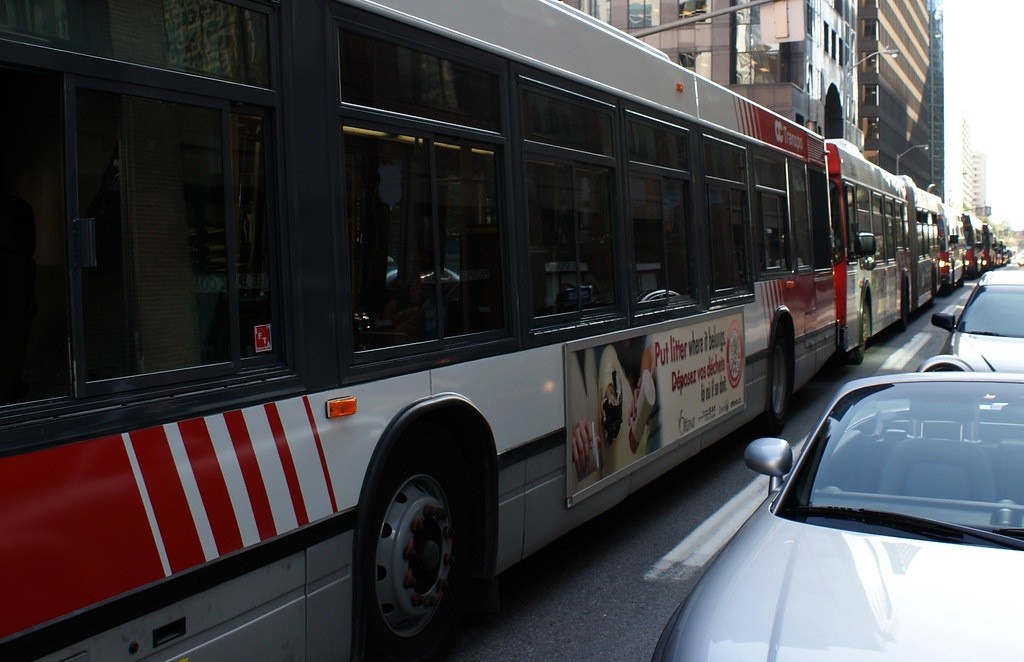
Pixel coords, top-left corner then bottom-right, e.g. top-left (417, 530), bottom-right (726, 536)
top-left (881, 438), bottom-right (995, 501)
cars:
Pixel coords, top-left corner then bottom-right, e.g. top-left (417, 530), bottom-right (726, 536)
top-left (931, 269), bottom-right (1024, 374)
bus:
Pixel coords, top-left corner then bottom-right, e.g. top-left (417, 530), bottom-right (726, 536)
top-left (827, 140), bottom-right (1009, 364)
top-left (0, 0), bottom-right (838, 662)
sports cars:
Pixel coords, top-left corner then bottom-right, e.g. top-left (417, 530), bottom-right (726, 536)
top-left (653, 355), bottom-right (1024, 661)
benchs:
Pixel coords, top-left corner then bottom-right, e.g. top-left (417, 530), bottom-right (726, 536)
top-left (909, 396), bottom-right (1023, 505)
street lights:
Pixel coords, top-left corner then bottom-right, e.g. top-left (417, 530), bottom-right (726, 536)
top-left (896, 142), bottom-right (929, 177)
top-left (842, 49), bottom-right (897, 142)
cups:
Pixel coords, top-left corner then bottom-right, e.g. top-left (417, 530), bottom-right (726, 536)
top-left (576, 421), bottom-right (599, 483)
top-left (628, 370), bottom-right (656, 454)
